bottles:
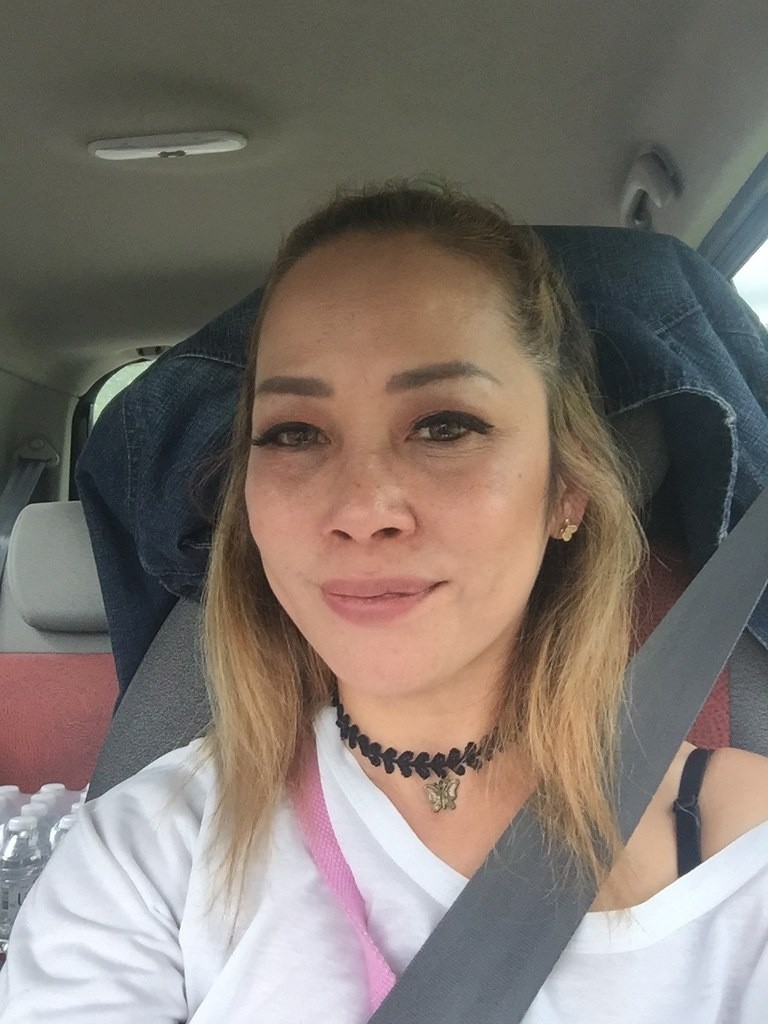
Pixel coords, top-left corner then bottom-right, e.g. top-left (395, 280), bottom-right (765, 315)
top-left (0, 783), bottom-right (90, 954)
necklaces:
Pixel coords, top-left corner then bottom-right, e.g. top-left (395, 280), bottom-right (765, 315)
top-left (332, 684), bottom-right (523, 811)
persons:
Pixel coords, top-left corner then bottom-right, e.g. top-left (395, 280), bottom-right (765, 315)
top-left (0, 184), bottom-right (768, 1024)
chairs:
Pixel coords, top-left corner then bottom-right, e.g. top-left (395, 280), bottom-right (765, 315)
top-left (0, 229), bottom-right (768, 961)
